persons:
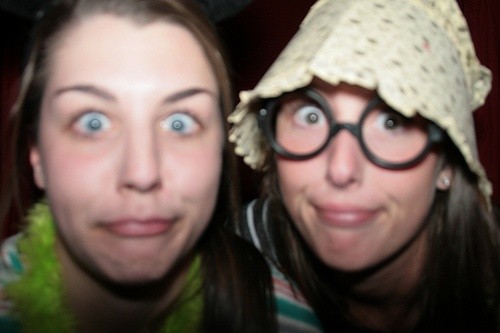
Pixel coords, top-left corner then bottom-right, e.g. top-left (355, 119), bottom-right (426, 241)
top-left (0, 0), bottom-right (320, 333)
top-left (224, 0), bottom-right (500, 333)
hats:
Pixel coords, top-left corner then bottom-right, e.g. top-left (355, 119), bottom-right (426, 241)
top-left (220, 1), bottom-right (492, 203)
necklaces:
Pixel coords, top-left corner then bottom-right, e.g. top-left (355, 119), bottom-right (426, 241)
top-left (10, 194), bottom-right (205, 333)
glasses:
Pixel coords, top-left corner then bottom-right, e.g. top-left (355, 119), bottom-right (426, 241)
top-left (255, 88), bottom-right (453, 170)
